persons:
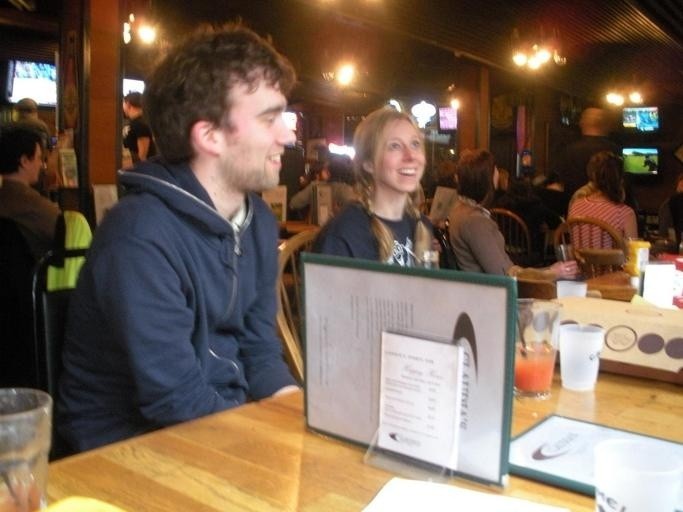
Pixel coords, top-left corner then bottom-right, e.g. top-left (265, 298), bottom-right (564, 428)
top-left (566, 149), bottom-right (639, 274)
top-left (311, 106), bottom-right (456, 272)
top-left (548, 106), bottom-right (619, 200)
top-left (47, 19), bottom-right (306, 466)
top-left (279, 146), bottom-right (568, 269)
top-left (1, 91), bottom-right (157, 396)
top-left (641, 153), bottom-right (658, 175)
top-left (444, 146), bottom-right (581, 284)
top-left (669, 144), bottom-right (683, 255)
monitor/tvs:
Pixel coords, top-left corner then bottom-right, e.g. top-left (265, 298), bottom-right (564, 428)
top-left (622, 107), bottom-right (659, 131)
top-left (436, 106), bottom-right (458, 133)
top-left (621, 146), bottom-right (661, 179)
top-left (123, 78), bottom-right (146, 100)
top-left (5, 57), bottom-right (57, 112)
top-left (283, 110), bottom-right (303, 131)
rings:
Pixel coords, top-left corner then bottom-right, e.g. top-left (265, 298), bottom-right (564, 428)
top-left (566, 267), bottom-right (571, 271)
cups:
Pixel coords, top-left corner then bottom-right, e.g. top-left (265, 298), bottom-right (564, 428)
top-left (590, 438), bottom-right (676, 511)
top-left (553, 243), bottom-right (580, 281)
top-left (516, 298), bottom-right (564, 402)
top-left (560, 324), bottom-right (605, 391)
top-left (626, 241), bottom-right (650, 277)
top-left (0, 387), bottom-right (53, 511)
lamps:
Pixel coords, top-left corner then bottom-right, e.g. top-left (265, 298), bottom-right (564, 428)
top-left (507, 23), bottom-right (566, 70)
top-left (520, 149), bottom-right (532, 173)
